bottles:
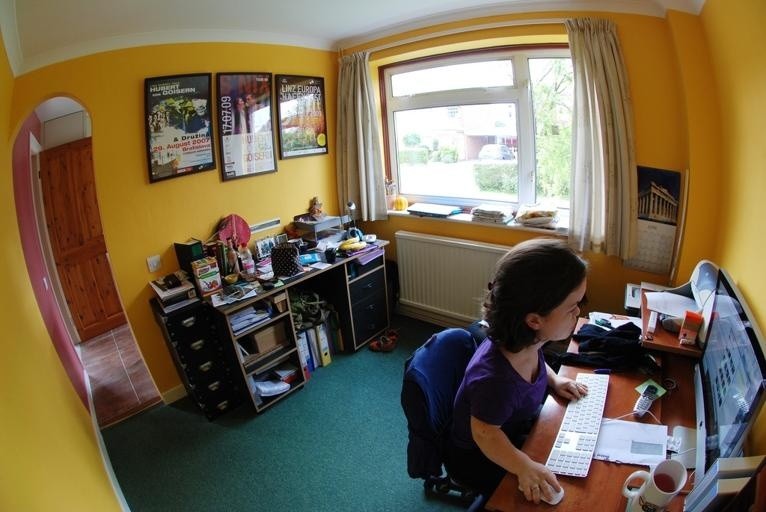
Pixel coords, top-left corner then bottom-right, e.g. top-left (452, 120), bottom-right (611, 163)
top-left (240, 242), bottom-right (257, 281)
top-left (226, 238), bottom-right (241, 275)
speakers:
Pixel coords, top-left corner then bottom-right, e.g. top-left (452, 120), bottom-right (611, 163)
top-left (685, 455), bottom-right (766, 512)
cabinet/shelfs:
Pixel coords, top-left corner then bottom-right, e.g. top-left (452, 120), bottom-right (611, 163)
top-left (146, 298), bottom-right (239, 422)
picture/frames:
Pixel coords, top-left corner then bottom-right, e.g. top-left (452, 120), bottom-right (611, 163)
top-left (215, 72), bottom-right (279, 182)
top-left (274, 73), bottom-right (328, 161)
top-left (144, 72), bottom-right (215, 184)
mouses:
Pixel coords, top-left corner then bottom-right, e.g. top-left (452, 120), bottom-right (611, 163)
top-left (519, 475), bottom-right (565, 506)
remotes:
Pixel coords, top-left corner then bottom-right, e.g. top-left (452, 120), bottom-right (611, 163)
top-left (633, 383), bottom-right (659, 418)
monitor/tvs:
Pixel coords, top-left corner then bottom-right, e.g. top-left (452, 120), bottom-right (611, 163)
top-left (694, 267), bottom-right (766, 482)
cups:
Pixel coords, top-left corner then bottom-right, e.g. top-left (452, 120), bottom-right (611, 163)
top-left (622, 460), bottom-right (688, 511)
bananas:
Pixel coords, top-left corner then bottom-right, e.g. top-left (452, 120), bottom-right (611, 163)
top-left (340, 238), bottom-right (368, 252)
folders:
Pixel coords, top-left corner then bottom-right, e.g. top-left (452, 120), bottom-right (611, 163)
top-left (315, 324), bottom-right (332, 368)
top-left (297, 336), bottom-right (311, 382)
top-left (307, 328), bottom-right (322, 369)
top-left (297, 331), bottom-right (315, 373)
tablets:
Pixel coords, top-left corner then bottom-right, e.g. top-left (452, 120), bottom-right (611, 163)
top-left (409, 202), bottom-right (457, 218)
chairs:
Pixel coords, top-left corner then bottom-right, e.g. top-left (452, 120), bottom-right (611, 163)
top-left (408, 328), bottom-right (480, 503)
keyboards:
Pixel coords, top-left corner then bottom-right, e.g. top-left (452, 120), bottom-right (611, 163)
top-left (542, 373), bottom-right (615, 478)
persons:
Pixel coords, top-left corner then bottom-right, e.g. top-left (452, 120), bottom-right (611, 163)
top-left (452, 236), bottom-right (589, 505)
top-left (231, 91), bottom-right (268, 136)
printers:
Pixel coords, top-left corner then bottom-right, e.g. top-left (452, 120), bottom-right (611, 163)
top-left (662, 259), bottom-right (719, 348)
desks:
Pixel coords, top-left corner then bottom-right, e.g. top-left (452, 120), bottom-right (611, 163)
top-left (200, 227), bottom-right (393, 416)
top-left (484, 288), bottom-right (702, 512)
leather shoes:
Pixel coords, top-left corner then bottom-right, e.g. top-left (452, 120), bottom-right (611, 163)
top-left (369, 336), bottom-right (394, 353)
top-left (385, 331), bottom-right (398, 347)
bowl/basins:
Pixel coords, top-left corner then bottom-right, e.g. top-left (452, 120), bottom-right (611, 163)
top-left (225, 273), bottom-right (239, 283)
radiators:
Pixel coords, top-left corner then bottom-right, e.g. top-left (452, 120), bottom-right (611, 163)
top-left (394, 231), bottom-right (515, 324)
